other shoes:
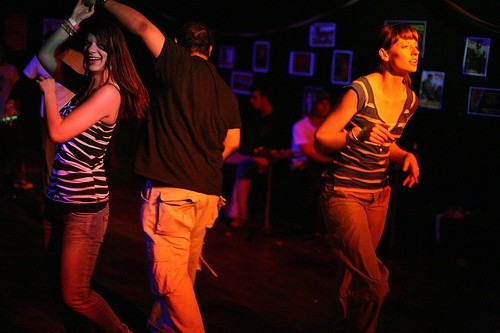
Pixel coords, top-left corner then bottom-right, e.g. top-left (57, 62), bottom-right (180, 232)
top-left (231, 218), bottom-right (245, 228)
top-left (13, 174), bottom-right (33, 194)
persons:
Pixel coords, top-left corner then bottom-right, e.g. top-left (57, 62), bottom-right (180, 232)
top-left (224, 80), bottom-right (334, 230)
top-left (421, 73), bottom-right (444, 101)
top-left (313, 21), bottom-right (420, 333)
top-left (36, 0), bottom-right (149, 333)
top-left (91, 0), bottom-right (242, 333)
top-left (0, 59), bottom-right (48, 184)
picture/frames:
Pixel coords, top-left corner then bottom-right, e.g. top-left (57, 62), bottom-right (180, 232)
top-left (218, 21), bottom-right (500, 119)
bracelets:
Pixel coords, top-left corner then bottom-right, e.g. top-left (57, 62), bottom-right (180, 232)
top-left (101, 0), bottom-right (107, 4)
top-left (61, 17), bottom-right (80, 36)
top-left (351, 126), bottom-right (371, 148)
